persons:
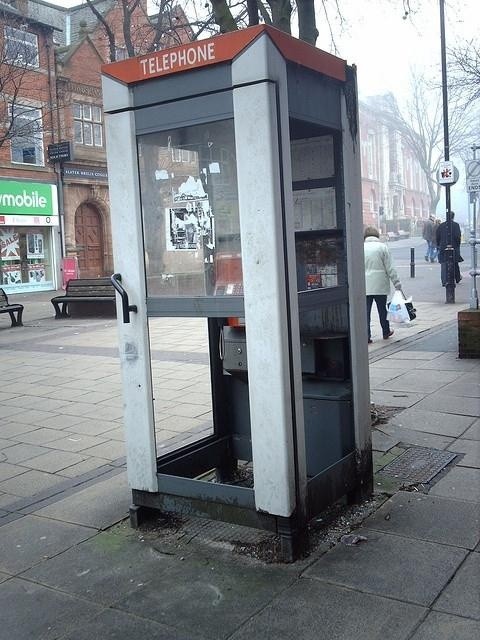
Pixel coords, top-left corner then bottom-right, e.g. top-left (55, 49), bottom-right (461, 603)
top-left (363, 225), bottom-right (405, 345)
top-left (422, 217), bottom-right (435, 262)
top-left (437, 210), bottom-right (464, 288)
top-left (429, 218), bottom-right (441, 264)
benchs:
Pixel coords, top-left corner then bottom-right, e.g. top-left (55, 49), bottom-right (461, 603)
top-left (51, 278), bottom-right (116, 319)
top-left (0, 288), bottom-right (23, 326)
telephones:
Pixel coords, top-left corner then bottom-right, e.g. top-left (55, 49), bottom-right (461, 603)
top-left (215, 252), bottom-right (246, 330)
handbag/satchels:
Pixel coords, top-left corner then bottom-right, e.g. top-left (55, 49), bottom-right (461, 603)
top-left (385, 288), bottom-right (416, 323)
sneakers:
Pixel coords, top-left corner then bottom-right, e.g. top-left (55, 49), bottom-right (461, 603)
top-left (368, 338), bottom-right (373, 343)
top-left (383, 329), bottom-right (394, 339)
